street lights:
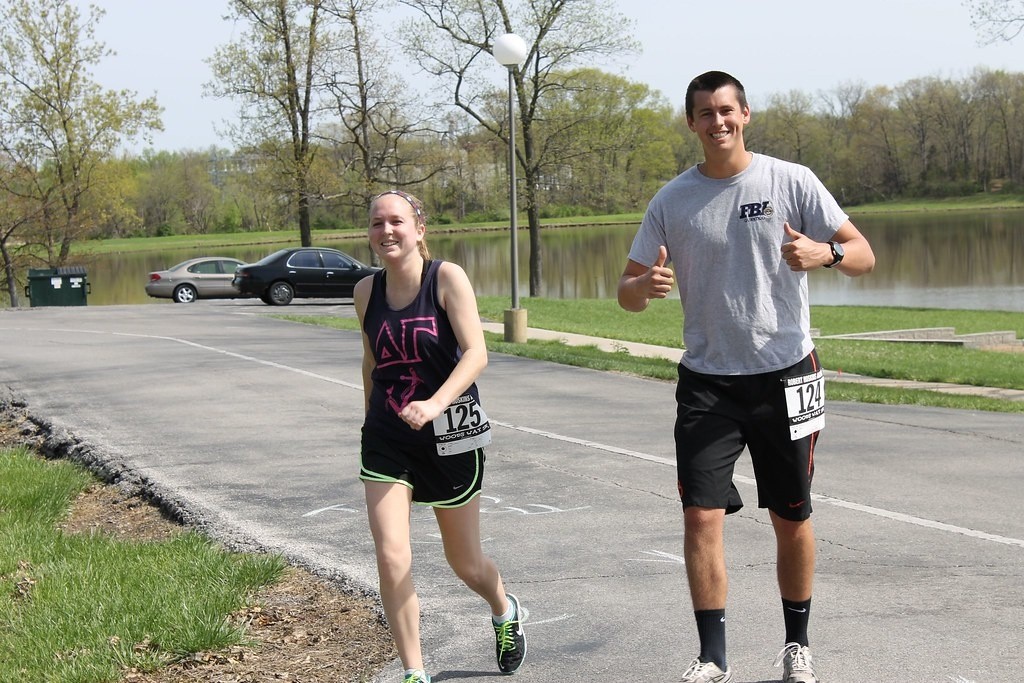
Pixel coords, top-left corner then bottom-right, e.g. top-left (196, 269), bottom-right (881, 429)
top-left (492, 33), bottom-right (528, 345)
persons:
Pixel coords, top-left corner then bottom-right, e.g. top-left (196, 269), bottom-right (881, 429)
top-left (353, 190), bottom-right (526, 683)
top-left (617, 70), bottom-right (876, 683)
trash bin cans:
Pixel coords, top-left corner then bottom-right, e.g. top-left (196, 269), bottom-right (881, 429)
top-left (25, 267), bottom-right (92, 307)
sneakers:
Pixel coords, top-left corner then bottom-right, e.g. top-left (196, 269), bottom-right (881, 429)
top-left (400, 669), bottom-right (431, 683)
top-left (678, 658), bottom-right (733, 683)
top-left (492, 593), bottom-right (527, 674)
top-left (772, 642), bottom-right (820, 683)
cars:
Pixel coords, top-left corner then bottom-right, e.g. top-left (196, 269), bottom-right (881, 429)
top-left (145, 256), bottom-right (250, 304)
top-left (231, 246), bottom-right (384, 306)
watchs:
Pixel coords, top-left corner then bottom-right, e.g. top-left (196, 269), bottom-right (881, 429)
top-left (822, 241), bottom-right (844, 268)
